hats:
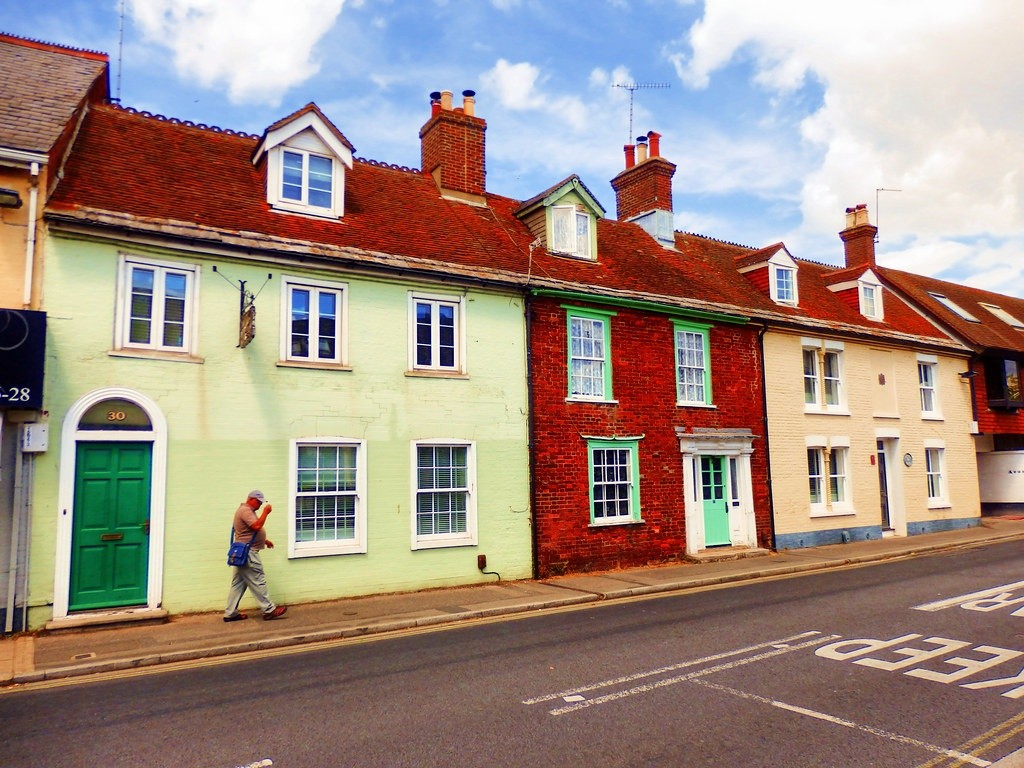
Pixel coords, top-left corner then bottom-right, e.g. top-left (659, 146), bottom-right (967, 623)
top-left (248, 490), bottom-right (268, 503)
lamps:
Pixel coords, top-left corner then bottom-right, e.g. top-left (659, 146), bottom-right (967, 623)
top-left (958, 368), bottom-right (979, 378)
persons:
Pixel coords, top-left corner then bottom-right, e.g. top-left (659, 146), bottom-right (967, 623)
top-left (223, 490), bottom-right (288, 622)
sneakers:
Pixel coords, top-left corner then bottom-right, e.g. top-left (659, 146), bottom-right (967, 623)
top-left (263, 606), bottom-right (287, 620)
top-left (224, 614), bottom-right (247, 622)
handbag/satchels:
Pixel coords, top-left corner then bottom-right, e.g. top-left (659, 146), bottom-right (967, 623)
top-left (227, 542), bottom-right (250, 566)
